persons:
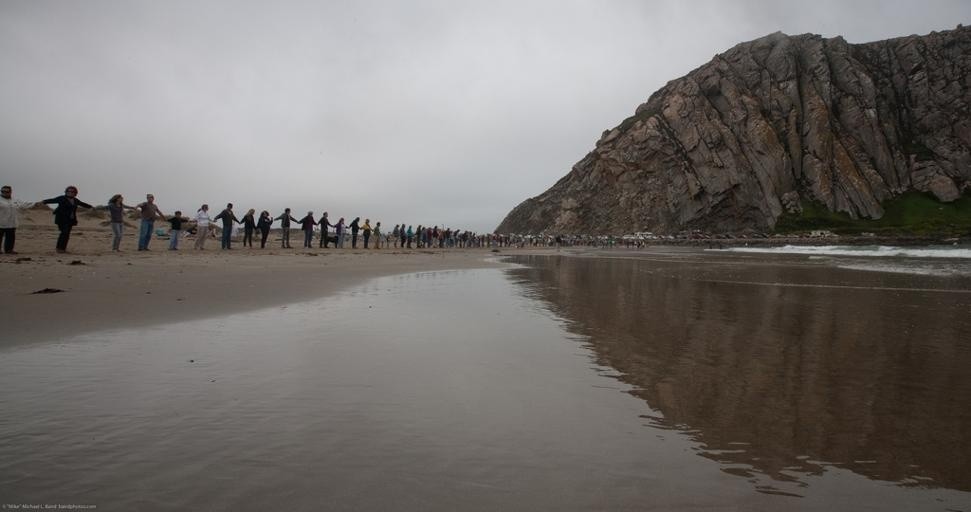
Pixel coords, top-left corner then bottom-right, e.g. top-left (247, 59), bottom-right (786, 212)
top-left (38, 181), bottom-right (95, 255)
top-left (274, 207), bottom-right (299, 251)
top-left (1, 185), bottom-right (41, 256)
top-left (240, 206), bottom-right (258, 249)
top-left (135, 194), bottom-right (168, 249)
top-left (318, 210), bottom-right (334, 249)
top-left (216, 203), bottom-right (240, 249)
top-left (168, 207), bottom-right (189, 252)
top-left (375, 220), bottom-right (647, 248)
top-left (298, 210), bottom-right (319, 249)
top-left (257, 210), bottom-right (275, 248)
top-left (334, 217), bottom-right (349, 248)
top-left (346, 216), bottom-right (362, 248)
top-left (189, 200), bottom-right (217, 251)
top-left (95, 190), bottom-right (137, 251)
top-left (358, 216), bottom-right (374, 247)
